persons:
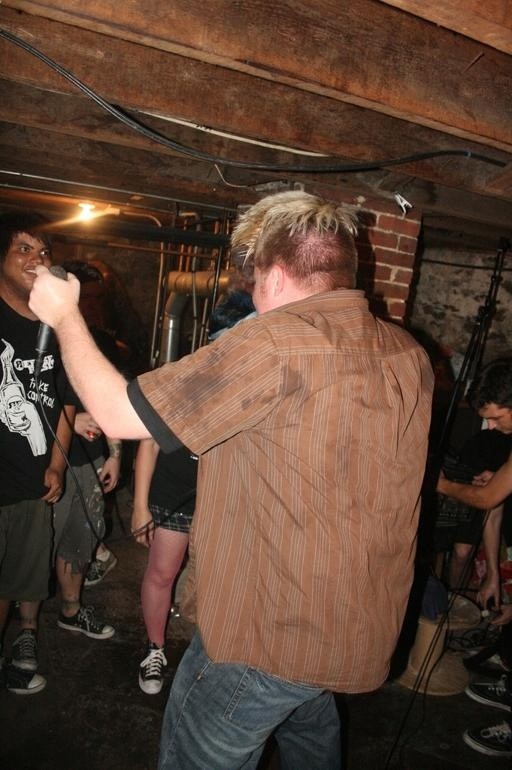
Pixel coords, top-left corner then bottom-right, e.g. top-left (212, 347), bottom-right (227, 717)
top-left (24, 184), bottom-right (434, 770)
top-left (12, 255), bottom-right (117, 672)
top-left (81, 541), bottom-right (121, 591)
top-left (435, 399), bottom-right (511, 681)
top-left (435, 355), bottom-right (511, 759)
top-left (128, 284), bottom-right (261, 694)
top-left (0, 209), bottom-right (78, 696)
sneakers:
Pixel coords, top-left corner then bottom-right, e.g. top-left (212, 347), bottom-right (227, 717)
top-left (57, 606), bottom-right (115, 640)
top-left (465, 677), bottom-right (512, 711)
top-left (139, 643), bottom-right (166, 694)
top-left (462, 720), bottom-right (511, 756)
top-left (1, 656), bottom-right (47, 695)
top-left (82, 549), bottom-right (117, 586)
top-left (12, 628), bottom-right (38, 670)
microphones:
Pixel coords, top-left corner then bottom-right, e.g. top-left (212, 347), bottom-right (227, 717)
top-left (33, 265), bottom-right (68, 376)
